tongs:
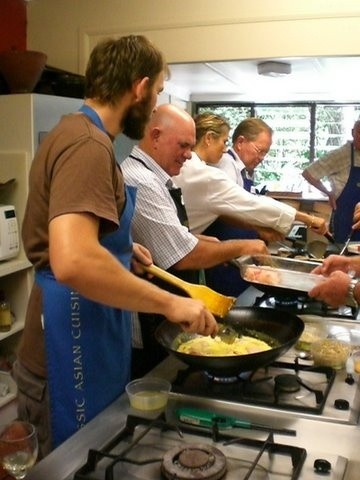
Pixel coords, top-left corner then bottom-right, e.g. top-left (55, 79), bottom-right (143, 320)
top-left (217, 322), bottom-right (236, 345)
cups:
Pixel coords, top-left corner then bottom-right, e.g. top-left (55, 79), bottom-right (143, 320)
top-left (0, 302), bottom-right (11, 332)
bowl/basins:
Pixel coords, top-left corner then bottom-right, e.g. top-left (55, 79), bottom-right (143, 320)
top-left (0, 50), bottom-right (47, 93)
top-left (126, 378), bottom-right (171, 409)
top-left (295, 323), bottom-right (329, 352)
top-left (310, 338), bottom-right (350, 369)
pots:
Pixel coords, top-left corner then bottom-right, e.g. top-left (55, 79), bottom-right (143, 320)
top-left (155, 305), bottom-right (304, 373)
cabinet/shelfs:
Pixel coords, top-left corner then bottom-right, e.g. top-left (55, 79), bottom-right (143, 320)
top-left (1, 263), bottom-right (35, 480)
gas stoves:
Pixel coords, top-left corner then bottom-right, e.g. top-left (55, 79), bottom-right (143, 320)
top-left (253, 294), bottom-right (360, 321)
top-left (71, 413), bottom-right (348, 480)
top-left (165, 360), bottom-right (360, 426)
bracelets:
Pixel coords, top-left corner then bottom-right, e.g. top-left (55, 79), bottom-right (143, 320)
top-left (308, 214), bottom-right (315, 229)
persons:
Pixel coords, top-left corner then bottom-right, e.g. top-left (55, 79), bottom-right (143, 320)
top-left (215, 117), bottom-right (285, 298)
top-left (10, 35), bottom-right (218, 460)
top-left (352, 202), bottom-right (360, 229)
top-left (302, 120), bottom-right (360, 244)
top-left (169, 111), bottom-right (332, 291)
top-left (120, 103), bottom-right (274, 382)
top-left (307, 254), bottom-right (360, 308)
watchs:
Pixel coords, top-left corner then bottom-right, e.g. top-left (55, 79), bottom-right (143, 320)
top-left (344, 277), bottom-right (360, 308)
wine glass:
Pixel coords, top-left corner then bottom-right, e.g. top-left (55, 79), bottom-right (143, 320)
top-left (0, 421), bottom-right (39, 480)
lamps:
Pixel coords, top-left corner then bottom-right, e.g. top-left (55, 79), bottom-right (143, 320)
top-left (258, 62), bottom-right (292, 79)
